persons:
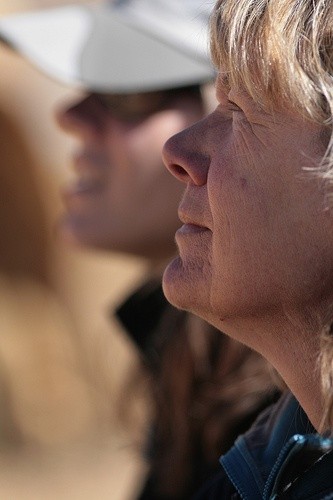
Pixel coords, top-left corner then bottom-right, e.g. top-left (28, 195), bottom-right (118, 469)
top-left (0, 0), bottom-right (288, 500)
top-left (158, 0), bottom-right (333, 499)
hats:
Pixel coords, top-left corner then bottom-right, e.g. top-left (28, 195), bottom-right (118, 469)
top-left (2, 0), bottom-right (220, 93)
